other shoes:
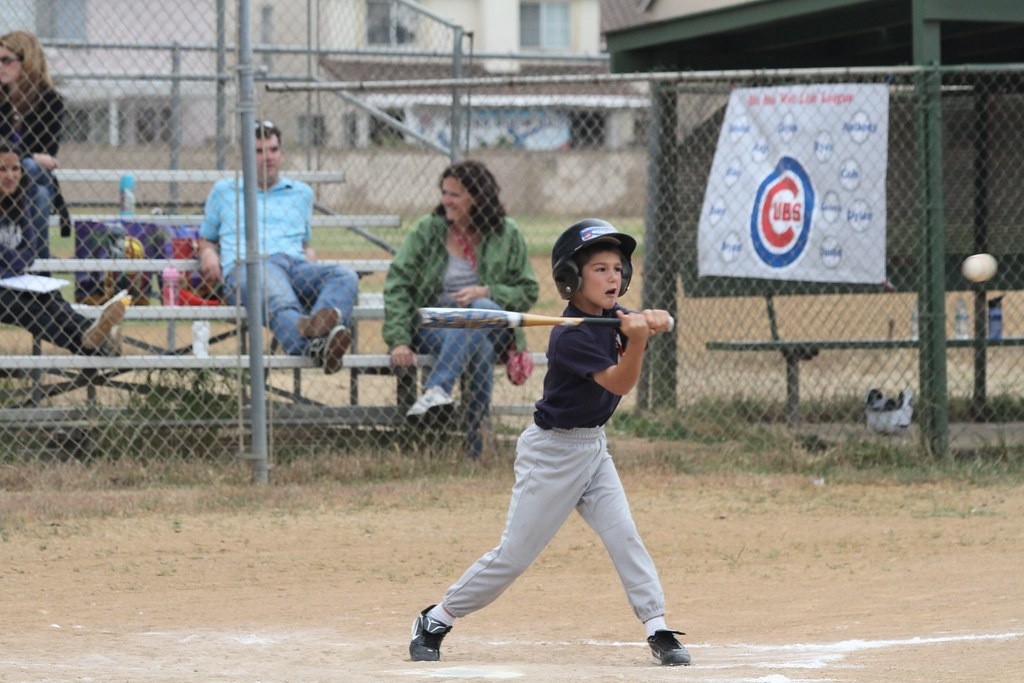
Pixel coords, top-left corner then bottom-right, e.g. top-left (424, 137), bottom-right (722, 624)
top-left (101, 326), bottom-right (122, 355)
top-left (321, 325), bottom-right (352, 374)
top-left (81, 299), bottom-right (127, 349)
top-left (298, 305), bottom-right (342, 337)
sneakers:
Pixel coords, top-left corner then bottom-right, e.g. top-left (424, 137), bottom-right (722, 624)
top-left (405, 386), bottom-right (454, 426)
top-left (409, 604), bottom-right (453, 661)
top-left (647, 629), bottom-right (690, 665)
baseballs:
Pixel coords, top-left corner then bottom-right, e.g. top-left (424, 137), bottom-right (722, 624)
top-left (962, 253), bottom-right (989, 282)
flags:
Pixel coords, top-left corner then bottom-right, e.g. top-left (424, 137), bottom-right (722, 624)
top-left (697, 83), bottom-right (889, 284)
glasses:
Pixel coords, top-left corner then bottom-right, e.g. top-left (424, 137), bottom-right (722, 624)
top-left (0, 55), bottom-right (23, 65)
top-left (256, 120), bottom-right (275, 131)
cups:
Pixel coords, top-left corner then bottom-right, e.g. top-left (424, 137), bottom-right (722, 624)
top-left (173, 237), bottom-right (193, 258)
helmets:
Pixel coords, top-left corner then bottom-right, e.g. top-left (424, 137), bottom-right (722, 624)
top-left (865, 387), bottom-right (912, 434)
top-left (551, 218), bottom-right (636, 300)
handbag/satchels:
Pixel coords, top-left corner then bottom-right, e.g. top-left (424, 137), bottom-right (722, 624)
top-left (506, 343), bottom-right (532, 384)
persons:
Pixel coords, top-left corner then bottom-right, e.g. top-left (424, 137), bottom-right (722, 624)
top-left (409, 218), bottom-right (691, 665)
top-left (0, 136), bottom-right (126, 373)
top-left (382, 160), bottom-right (539, 475)
top-left (0, 31), bottom-right (126, 253)
top-left (198, 120), bottom-right (359, 375)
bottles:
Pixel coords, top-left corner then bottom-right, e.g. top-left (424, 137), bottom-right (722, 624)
top-left (988, 294), bottom-right (1005, 338)
top-left (118, 175), bottom-right (135, 214)
top-left (161, 265), bottom-right (185, 306)
top-left (192, 320), bottom-right (209, 354)
top-left (912, 299), bottom-right (920, 339)
top-left (955, 295), bottom-right (970, 339)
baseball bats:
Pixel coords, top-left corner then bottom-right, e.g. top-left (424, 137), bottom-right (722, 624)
top-left (417, 306), bottom-right (676, 334)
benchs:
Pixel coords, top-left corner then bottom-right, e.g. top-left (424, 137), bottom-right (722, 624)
top-left (707, 337), bottom-right (1024, 427)
top-left (0, 169), bottom-right (554, 455)
